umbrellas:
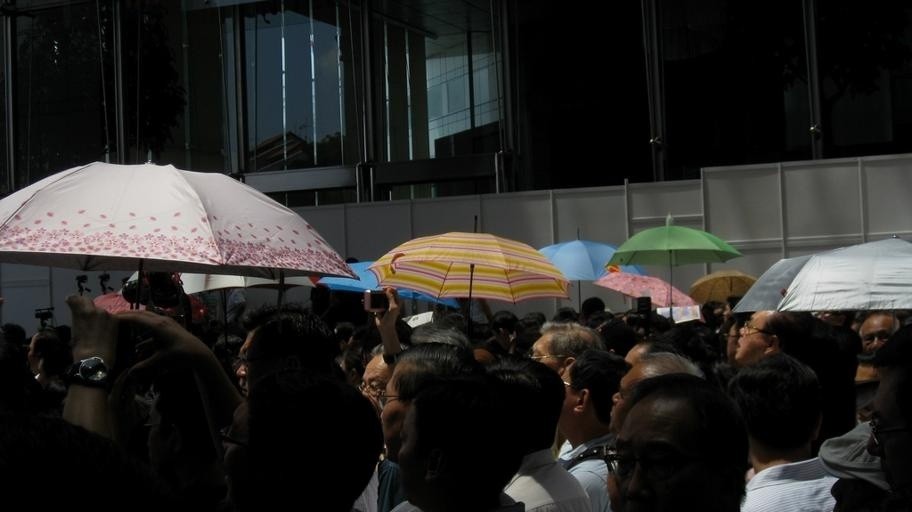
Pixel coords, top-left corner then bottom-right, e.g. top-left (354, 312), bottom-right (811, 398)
top-left (686, 269), bottom-right (759, 303)
top-left (365, 215), bottom-right (573, 321)
top-left (604, 214), bottom-right (739, 322)
top-left (596, 274), bottom-right (693, 308)
top-left (1, 149), bottom-right (360, 309)
top-left (117, 271), bottom-right (317, 359)
top-left (731, 238), bottom-right (912, 314)
top-left (314, 261), bottom-right (462, 309)
top-left (539, 226), bottom-right (648, 312)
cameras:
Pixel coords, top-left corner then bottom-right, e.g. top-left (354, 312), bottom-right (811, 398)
top-left (365, 290), bottom-right (388, 313)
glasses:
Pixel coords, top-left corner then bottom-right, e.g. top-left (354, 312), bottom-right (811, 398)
top-left (527, 350), bottom-right (569, 366)
top-left (356, 378), bottom-right (401, 410)
top-left (868, 412), bottom-right (912, 448)
top-left (743, 318), bottom-right (770, 338)
